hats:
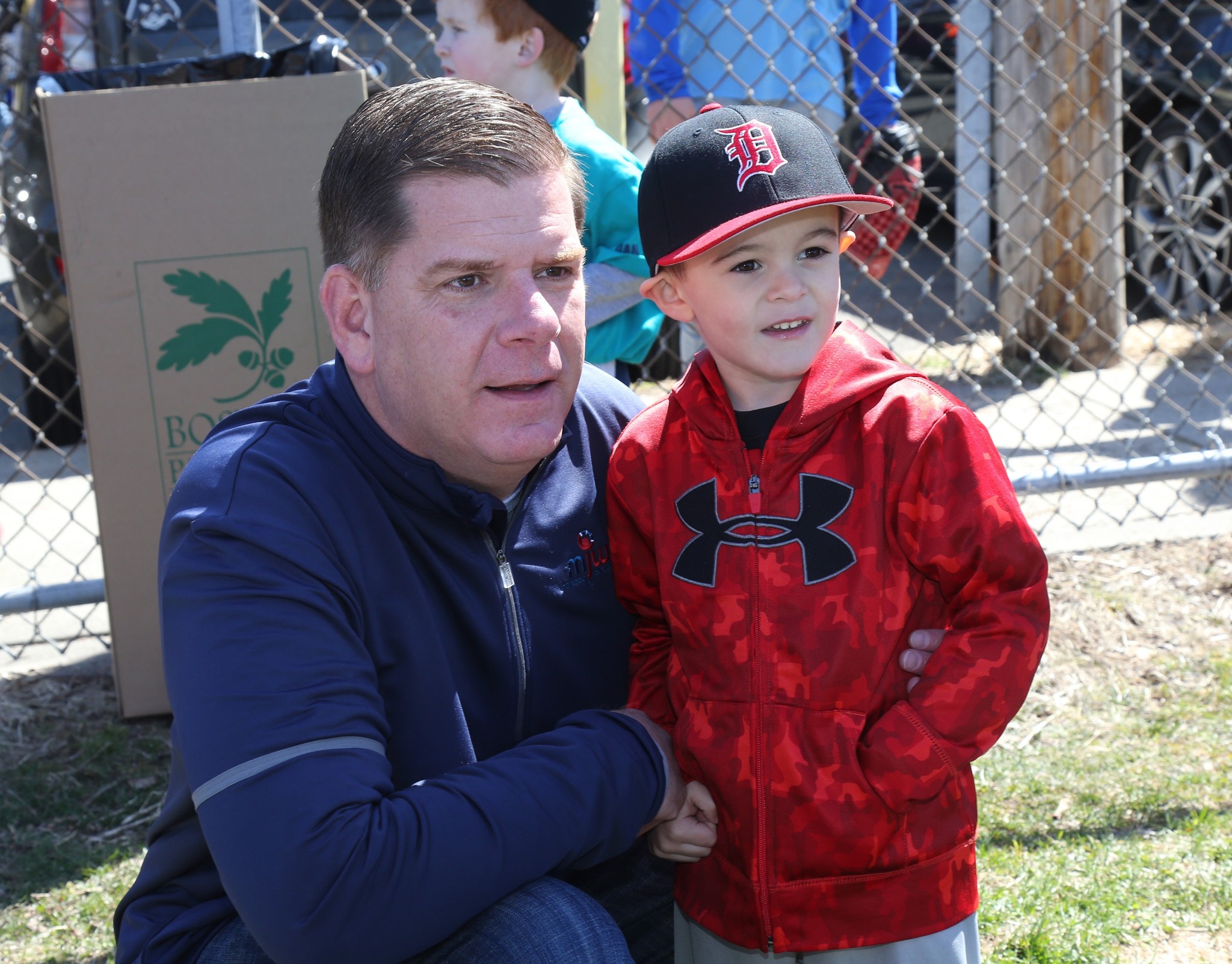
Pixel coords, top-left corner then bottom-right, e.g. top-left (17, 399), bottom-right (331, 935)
top-left (638, 103), bottom-right (893, 277)
top-left (523, 1), bottom-right (599, 54)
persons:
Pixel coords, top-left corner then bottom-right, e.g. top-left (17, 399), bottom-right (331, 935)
top-left (603, 101), bottom-right (1050, 964)
top-left (434, 0), bottom-right (665, 378)
top-left (112, 74), bottom-right (946, 964)
top-left (630, 0), bottom-right (923, 275)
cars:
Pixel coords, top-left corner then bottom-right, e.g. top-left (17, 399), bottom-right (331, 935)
top-left (896, 0), bottom-right (1231, 335)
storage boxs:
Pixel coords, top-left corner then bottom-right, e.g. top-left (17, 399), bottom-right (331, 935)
top-left (38, 69), bottom-right (366, 720)
